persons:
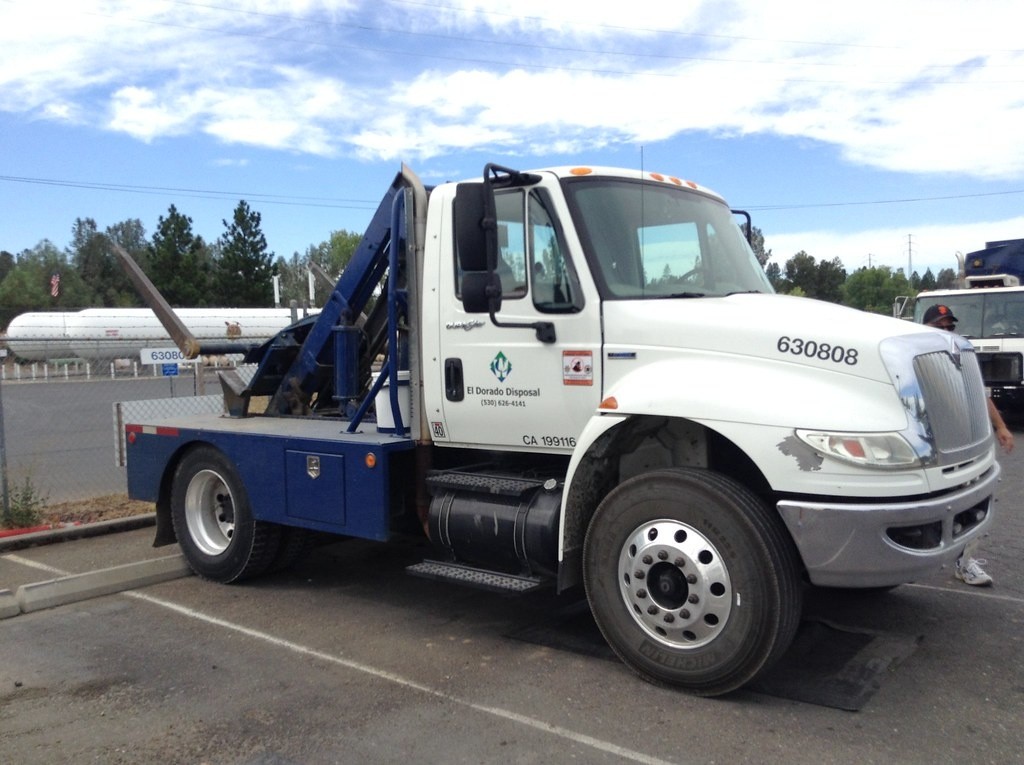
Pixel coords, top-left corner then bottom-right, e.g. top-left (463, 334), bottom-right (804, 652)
top-left (914, 303), bottom-right (1015, 587)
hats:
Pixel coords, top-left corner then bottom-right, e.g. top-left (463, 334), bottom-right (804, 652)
top-left (922, 304), bottom-right (958, 326)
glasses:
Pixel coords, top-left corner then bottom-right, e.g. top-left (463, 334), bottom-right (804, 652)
top-left (934, 324), bottom-right (955, 331)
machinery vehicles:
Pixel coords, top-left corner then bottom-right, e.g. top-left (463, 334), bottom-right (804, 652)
top-left (914, 240), bottom-right (1023, 433)
top-left (107, 161), bottom-right (1002, 700)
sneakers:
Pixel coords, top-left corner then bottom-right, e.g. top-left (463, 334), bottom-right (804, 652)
top-left (954, 555), bottom-right (994, 586)
